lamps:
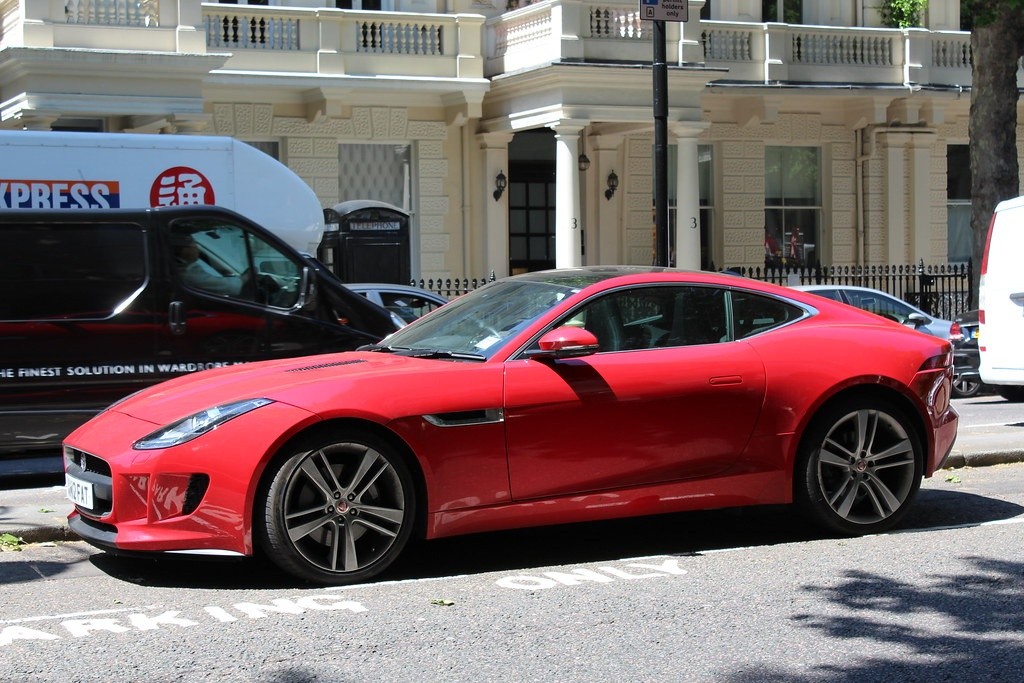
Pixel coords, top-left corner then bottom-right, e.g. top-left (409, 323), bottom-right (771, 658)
top-left (493, 170), bottom-right (507, 201)
top-left (578, 129), bottom-right (591, 171)
top-left (604, 170), bottom-right (618, 201)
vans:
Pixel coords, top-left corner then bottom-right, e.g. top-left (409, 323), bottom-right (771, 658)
top-left (975, 193), bottom-right (1024, 405)
top-left (0, 205), bottom-right (421, 483)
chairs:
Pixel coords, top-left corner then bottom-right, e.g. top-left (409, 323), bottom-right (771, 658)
top-left (584, 295), bottom-right (626, 351)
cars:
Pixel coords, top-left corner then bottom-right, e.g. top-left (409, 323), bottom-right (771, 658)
top-left (340, 281), bottom-right (451, 323)
top-left (63, 266), bottom-right (958, 585)
top-left (788, 283), bottom-right (983, 401)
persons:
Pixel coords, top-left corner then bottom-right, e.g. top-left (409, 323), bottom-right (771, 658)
top-left (166, 227), bottom-right (251, 294)
